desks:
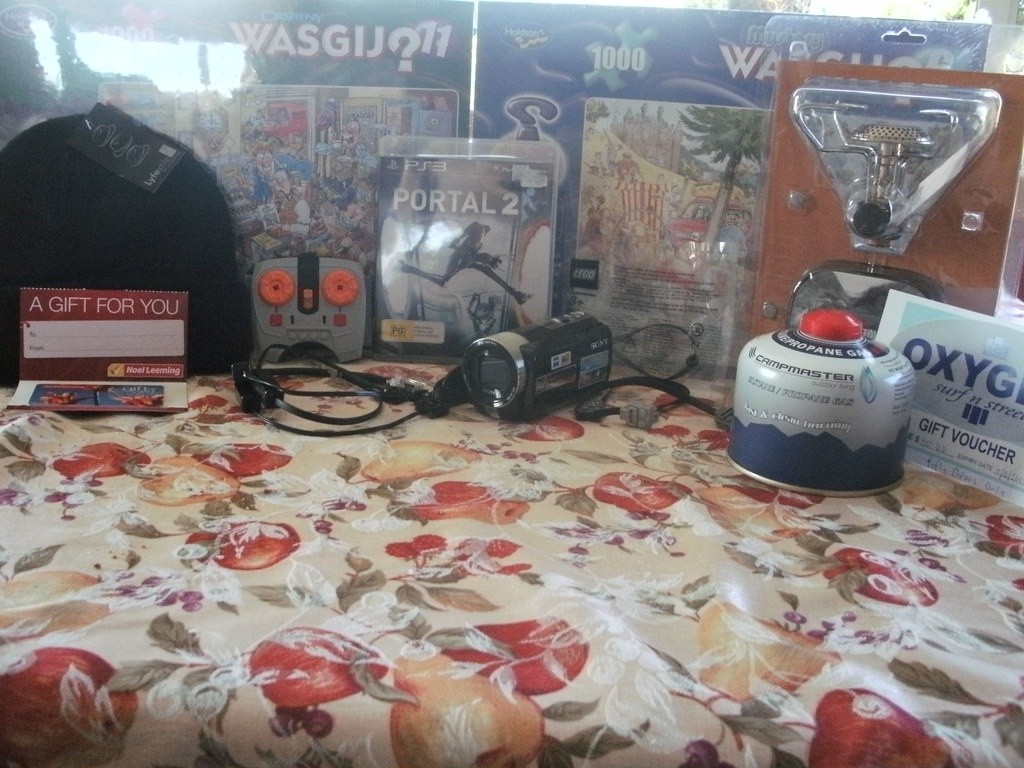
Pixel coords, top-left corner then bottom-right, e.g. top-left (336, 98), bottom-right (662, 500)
top-left (1, 354), bottom-right (1024, 768)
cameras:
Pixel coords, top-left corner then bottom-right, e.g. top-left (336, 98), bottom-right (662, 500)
top-left (459, 311), bottom-right (613, 423)
